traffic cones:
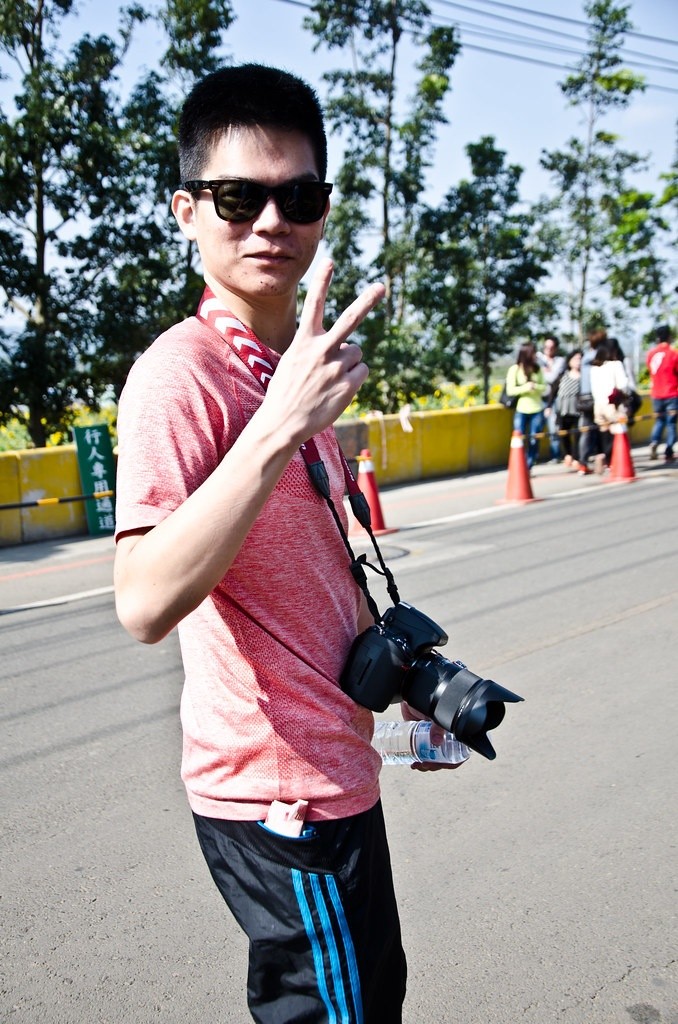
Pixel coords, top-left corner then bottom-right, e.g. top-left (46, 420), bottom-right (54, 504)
top-left (496, 430), bottom-right (545, 504)
top-left (600, 417), bottom-right (640, 482)
top-left (347, 449), bottom-right (400, 537)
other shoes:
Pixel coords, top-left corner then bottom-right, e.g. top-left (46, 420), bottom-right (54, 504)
top-left (651, 444), bottom-right (656, 459)
top-left (666, 453), bottom-right (678, 460)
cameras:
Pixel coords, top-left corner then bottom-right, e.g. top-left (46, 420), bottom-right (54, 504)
top-left (338, 602), bottom-right (524, 761)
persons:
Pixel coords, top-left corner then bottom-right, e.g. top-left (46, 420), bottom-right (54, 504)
top-left (544, 349), bottom-right (583, 468)
top-left (539, 337), bottom-right (565, 464)
top-left (645, 325), bottom-right (678, 464)
top-left (110, 64), bottom-right (406, 1024)
top-left (509, 342), bottom-right (552, 471)
top-left (587, 338), bottom-right (630, 471)
top-left (577, 332), bottom-right (607, 474)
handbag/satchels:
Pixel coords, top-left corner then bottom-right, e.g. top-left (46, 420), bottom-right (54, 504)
top-left (615, 391), bottom-right (642, 417)
top-left (500, 364), bottom-right (520, 409)
top-left (577, 395), bottom-right (594, 411)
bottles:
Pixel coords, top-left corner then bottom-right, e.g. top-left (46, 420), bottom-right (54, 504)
top-left (369, 722), bottom-right (473, 765)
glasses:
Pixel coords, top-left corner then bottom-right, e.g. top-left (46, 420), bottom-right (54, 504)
top-left (185, 180), bottom-right (334, 225)
